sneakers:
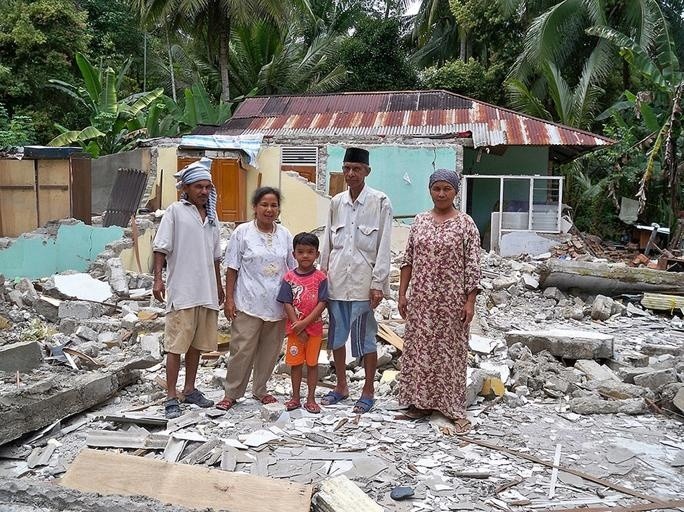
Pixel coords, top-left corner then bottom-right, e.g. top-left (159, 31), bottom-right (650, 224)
top-left (179, 388), bottom-right (214, 407)
top-left (164, 399), bottom-right (181, 419)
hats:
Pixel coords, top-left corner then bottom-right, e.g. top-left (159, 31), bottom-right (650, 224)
top-left (344, 148), bottom-right (369, 165)
top-left (429, 169), bottom-right (459, 194)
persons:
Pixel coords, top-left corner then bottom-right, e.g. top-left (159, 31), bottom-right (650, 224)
top-left (277, 231), bottom-right (327, 413)
top-left (397, 168), bottom-right (482, 436)
top-left (317, 146), bottom-right (393, 413)
top-left (151, 158), bottom-right (225, 418)
top-left (215, 186), bottom-right (299, 411)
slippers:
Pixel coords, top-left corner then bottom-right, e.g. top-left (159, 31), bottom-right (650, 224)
top-left (253, 393), bottom-right (279, 405)
top-left (321, 390), bottom-right (349, 406)
top-left (216, 400), bottom-right (236, 411)
top-left (405, 408), bottom-right (425, 419)
top-left (304, 403), bottom-right (321, 413)
top-left (285, 399), bottom-right (302, 411)
top-left (352, 398), bottom-right (374, 413)
top-left (456, 422), bottom-right (471, 435)
